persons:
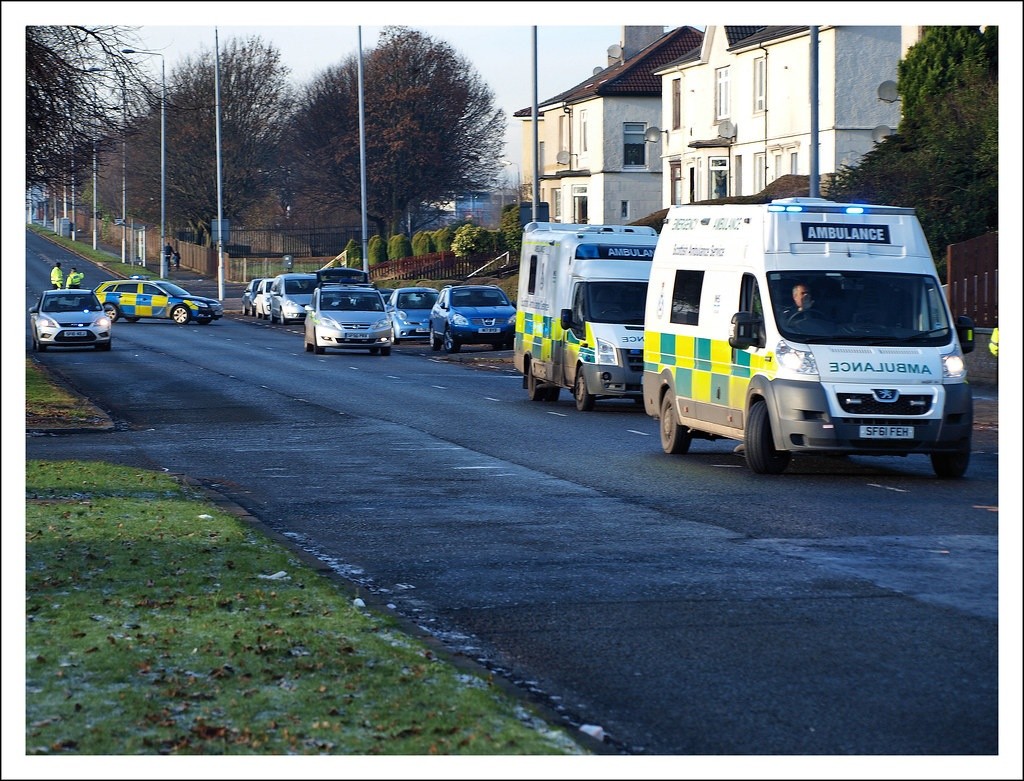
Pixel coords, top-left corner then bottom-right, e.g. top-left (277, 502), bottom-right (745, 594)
top-left (66, 267), bottom-right (85, 289)
top-left (50, 262), bottom-right (63, 290)
top-left (988, 327), bottom-right (999, 357)
top-left (172, 250), bottom-right (181, 267)
top-left (780, 285), bottom-right (829, 321)
top-left (165, 242), bottom-right (174, 270)
top-left (70, 222), bottom-right (73, 231)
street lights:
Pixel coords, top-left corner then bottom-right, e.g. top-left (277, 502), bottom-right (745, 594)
top-left (121, 49), bottom-right (166, 279)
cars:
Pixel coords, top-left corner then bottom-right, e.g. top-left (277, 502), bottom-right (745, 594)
top-left (254, 278), bottom-right (303, 320)
top-left (304, 266), bottom-right (396, 356)
top-left (113, 215), bottom-right (126, 226)
top-left (430, 285), bottom-right (516, 354)
top-left (241, 278), bottom-right (271, 317)
top-left (92, 274), bottom-right (225, 326)
top-left (28, 288), bottom-right (113, 352)
top-left (270, 272), bottom-right (318, 326)
top-left (383, 287), bottom-right (440, 345)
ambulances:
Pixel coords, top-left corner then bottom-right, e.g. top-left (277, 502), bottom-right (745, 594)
top-left (642, 193), bottom-right (976, 480)
top-left (514, 223), bottom-right (661, 412)
